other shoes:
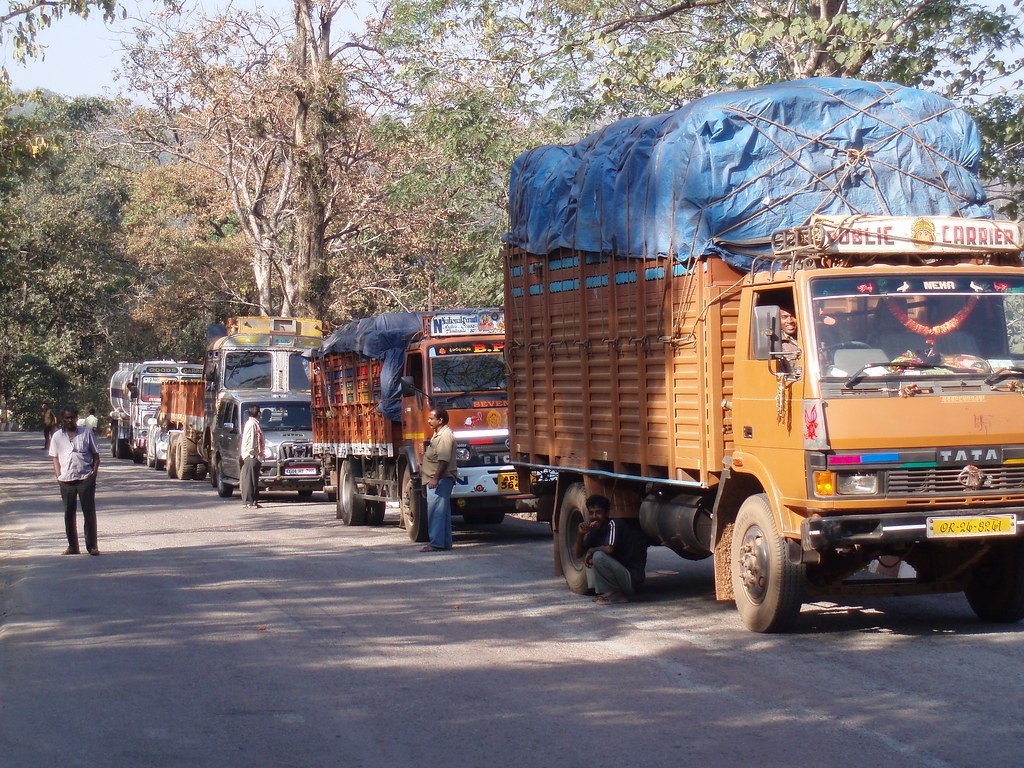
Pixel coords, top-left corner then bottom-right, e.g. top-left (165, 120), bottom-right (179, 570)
top-left (90, 547), bottom-right (99, 555)
top-left (60, 548), bottom-right (81, 555)
top-left (246, 503), bottom-right (261, 509)
top-left (419, 545), bottom-right (452, 552)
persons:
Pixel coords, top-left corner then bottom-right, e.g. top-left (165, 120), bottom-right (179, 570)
top-left (240, 405), bottom-right (264, 508)
top-left (780, 307), bottom-right (797, 372)
top-left (571, 493), bottom-right (647, 604)
top-left (41, 403), bottom-right (101, 555)
top-left (420, 407), bottom-right (457, 551)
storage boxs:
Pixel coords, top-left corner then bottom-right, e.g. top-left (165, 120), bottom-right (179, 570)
top-left (499, 238), bottom-right (742, 488)
top-left (309, 343), bottom-right (421, 457)
top-left (160, 380), bottom-right (205, 432)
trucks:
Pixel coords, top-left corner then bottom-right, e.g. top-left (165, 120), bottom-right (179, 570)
top-left (108, 316), bottom-right (328, 505)
top-left (501, 77), bottom-right (1024, 636)
top-left (318, 304), bottom-right (533, 544)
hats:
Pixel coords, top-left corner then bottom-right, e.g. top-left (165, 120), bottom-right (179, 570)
top-left (41, 404), bottom-right (47, 408)
